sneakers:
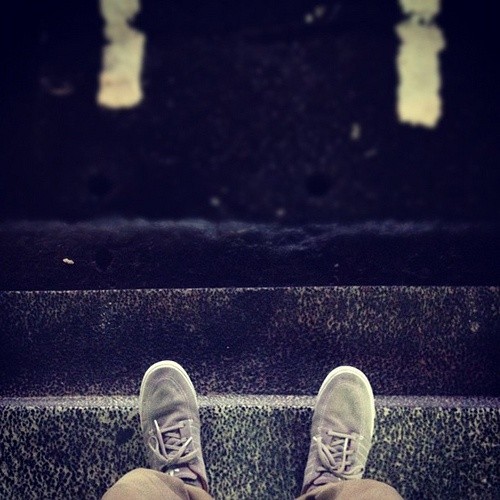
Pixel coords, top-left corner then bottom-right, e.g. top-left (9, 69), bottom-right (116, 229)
top-left (139, 360), bottom-right (208, 493)
top-left (300, 365), bottom-right (375, 495)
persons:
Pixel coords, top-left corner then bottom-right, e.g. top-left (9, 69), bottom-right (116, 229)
top-left (100, 359), bottom-right (407, 500)
